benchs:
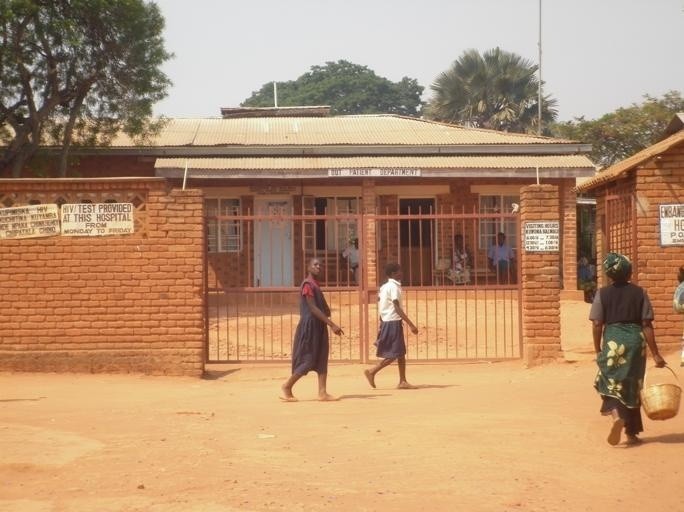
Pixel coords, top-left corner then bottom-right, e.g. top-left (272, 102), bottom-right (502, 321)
top-left (434, 259), bottom-right (513, 286)
top-left (304, 249), bottom-right (354, 285)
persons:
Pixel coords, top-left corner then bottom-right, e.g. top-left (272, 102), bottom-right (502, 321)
top-left (364, 261), bottom-right (418, 390)
top-left (278, 259), bottom-right (345, 401)
top-left (446, 234), bottom-right (471, 285)
top-left (577, 257), bottom-right (596, 302)
top-left (588, 253), bottom-right (664, 447)
top-left (342, 238), bottom-right (359, 281)
top-left (673, 266), bottom-right (684, 366)
top-left (489, 232), bottom-right (517, 284)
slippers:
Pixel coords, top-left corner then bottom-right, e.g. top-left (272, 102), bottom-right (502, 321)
top-left (279, 396), bottom-right (298, 402)
top-left (365, 370), bottom-right (375, 388)
top-left (318, 396), bottom-right (341, 401)
top-left (607, 418), bottom-right (642, 445)
top-left (399, 384), bottom-right (416, 388)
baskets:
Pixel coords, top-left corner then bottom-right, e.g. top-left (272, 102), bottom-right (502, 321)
top-left (640, 366), bottom-right (682, 419)
